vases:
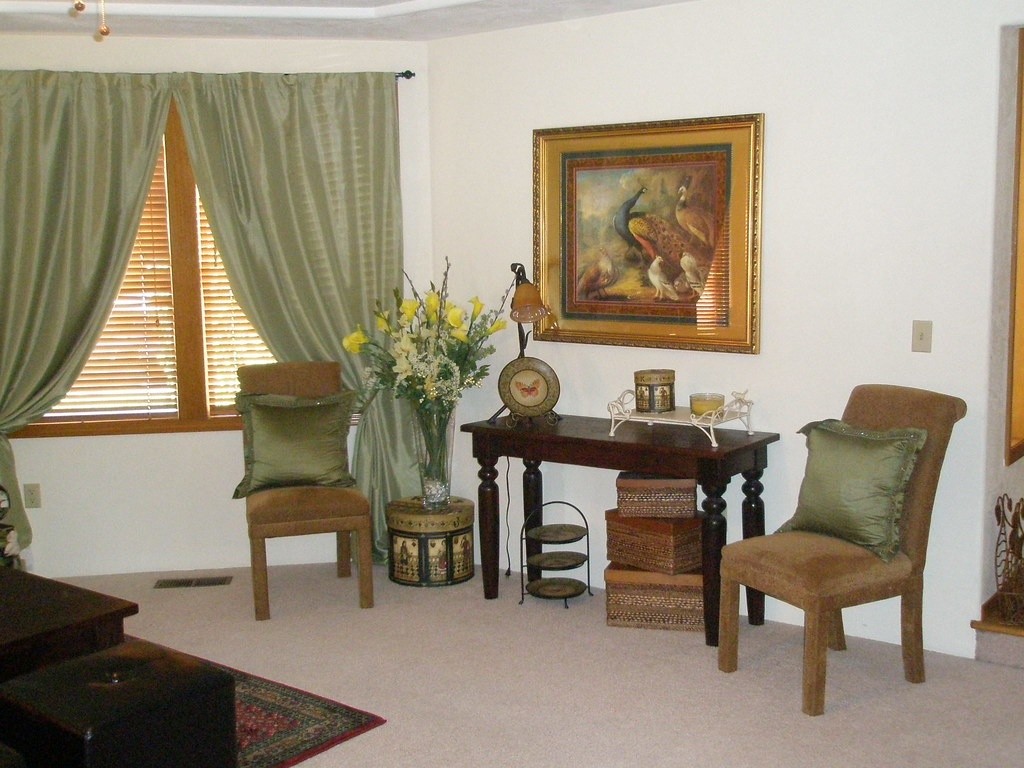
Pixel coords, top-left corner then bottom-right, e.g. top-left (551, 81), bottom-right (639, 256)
top-left (408, 400), bottom-right (456, 512)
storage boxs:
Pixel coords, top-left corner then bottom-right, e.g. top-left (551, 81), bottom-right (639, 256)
top-left (604, 562), bottom-right (706, 631)
top-left (616, 472), bottom-right (697, 519)
top-left (605, 509), bottom-right (705, 575)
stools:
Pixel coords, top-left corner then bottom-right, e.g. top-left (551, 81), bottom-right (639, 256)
top-left (0, 641), bottom-right (241, 768)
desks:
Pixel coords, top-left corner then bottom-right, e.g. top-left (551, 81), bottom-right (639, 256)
top-left (386, 494), bottom-right (475, 587)
top-left (0, 564), bottom-right (139, 683)
top-left (460, 412), bottom-right (780, 646)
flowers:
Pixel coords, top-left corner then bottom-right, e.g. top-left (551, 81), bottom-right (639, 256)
top-left (337, 255), bottom-right (517, 415)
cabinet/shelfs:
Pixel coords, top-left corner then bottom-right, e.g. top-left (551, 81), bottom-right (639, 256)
top-left (518, 501), bottom-right (594, 609)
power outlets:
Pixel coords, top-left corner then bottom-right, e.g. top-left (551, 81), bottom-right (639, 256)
top-left (236, 362), bottom-right (375, 621)
top-left (23, 484), bottom-right (42, 508)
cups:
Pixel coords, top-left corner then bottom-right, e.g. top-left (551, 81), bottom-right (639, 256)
top-left (689, 393), bottom-right (724, 419)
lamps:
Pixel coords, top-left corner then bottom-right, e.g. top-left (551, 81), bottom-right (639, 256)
top-left (487, 262), bottom-right (563, 425)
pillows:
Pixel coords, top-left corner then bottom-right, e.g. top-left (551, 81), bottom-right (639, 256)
top-left (775, 417), bottom-right (928, 567)
top-left (232, 392), bottom-right (357, 500)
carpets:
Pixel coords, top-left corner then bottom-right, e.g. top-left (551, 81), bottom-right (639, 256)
top-left (124, 635), bottom-right (386, 768)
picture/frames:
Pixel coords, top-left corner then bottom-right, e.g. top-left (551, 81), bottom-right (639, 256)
top-left (530, 113), bottom-right (764, 354)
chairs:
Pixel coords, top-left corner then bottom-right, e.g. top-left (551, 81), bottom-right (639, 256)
top-left (720, 383), bottom-right (968, 717)
